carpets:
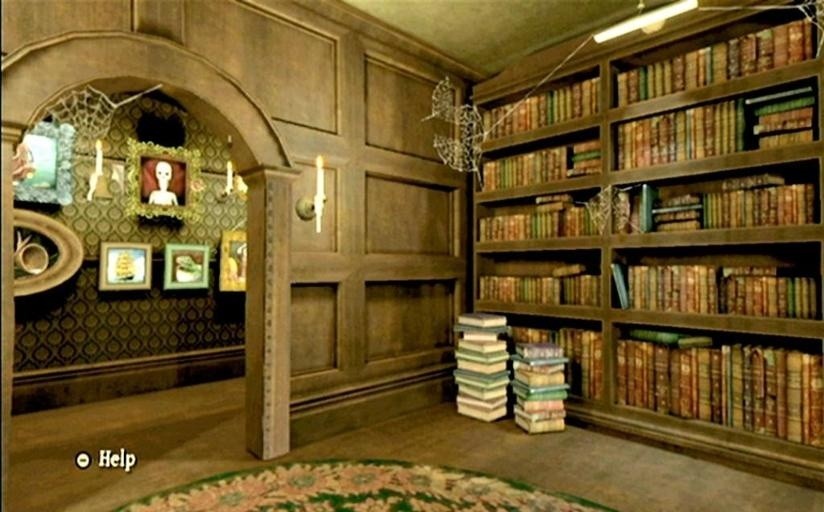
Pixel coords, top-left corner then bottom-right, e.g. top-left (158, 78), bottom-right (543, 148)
top-left (105, 459), bottom-right (601, 511)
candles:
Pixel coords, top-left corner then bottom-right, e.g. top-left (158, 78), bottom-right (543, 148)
top-left (315, 155), bottom-right (325, 205)
top-left (225, 161), bottom-right (232, 193)
top-left (94, 140), bottom-right (104, 173)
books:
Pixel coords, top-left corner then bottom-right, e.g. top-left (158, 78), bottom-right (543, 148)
top-left (449, 15), bottom-right (823, 449)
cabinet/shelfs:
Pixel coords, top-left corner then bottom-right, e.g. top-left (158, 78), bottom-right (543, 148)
top-left (471, 13), bottom-right (824, 494)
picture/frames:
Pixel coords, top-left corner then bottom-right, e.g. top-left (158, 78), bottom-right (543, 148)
top-left (126, 138), bottom-right (193, 221)
top-left (14, 121), bottom-right (76, 207)
top-left (97, 230), bottom-right (246, 292)
